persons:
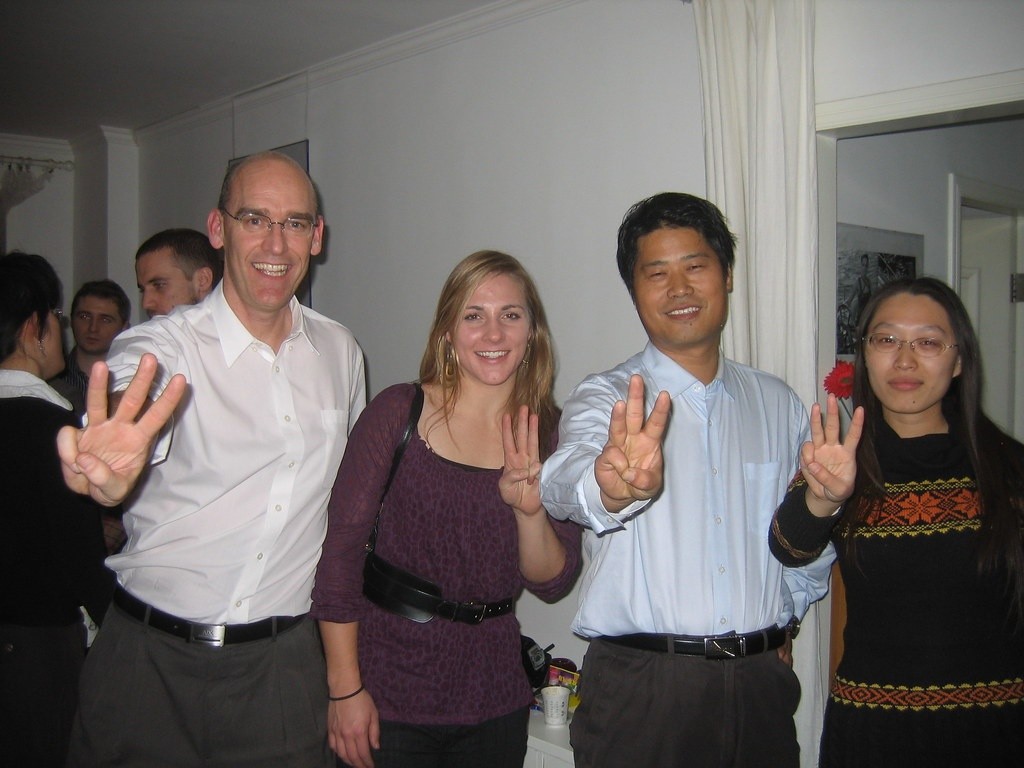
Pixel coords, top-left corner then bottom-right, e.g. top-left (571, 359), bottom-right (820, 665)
top-left (57, 152), bottom-right (367, 768)
top-left (309, 249), bottom-right (584, 768)
top-left (539, 192), bottom-right (836, 768)
top-left (768, 276), bottom-right (1024, 768)
top-left (1, 227), bottom-right (225, 768)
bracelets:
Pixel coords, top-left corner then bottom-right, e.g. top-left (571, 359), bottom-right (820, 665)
top-left (327, 684), bottom-right (365, 701)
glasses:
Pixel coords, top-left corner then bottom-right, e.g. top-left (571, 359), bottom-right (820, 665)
top-left (41, 303), bottom-right (63, 320)
top-left (862, 333), bottom-right (957, 358)
top-left (222, 207), bottom-right (318, 237)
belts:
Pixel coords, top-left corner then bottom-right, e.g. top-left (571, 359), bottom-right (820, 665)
top-left (599, 624), bottom-right (788, 661)
top-left (363, 555), bottom-right (514, 625)
top-left (113, 580), bottom-right (307, 647)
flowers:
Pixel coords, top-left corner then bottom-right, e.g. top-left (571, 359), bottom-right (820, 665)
top-left (822, 361), bottom-right (855, 421)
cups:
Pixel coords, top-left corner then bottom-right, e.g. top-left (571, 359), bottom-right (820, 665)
top-left (541, 686), bottom-right (570, 724)
